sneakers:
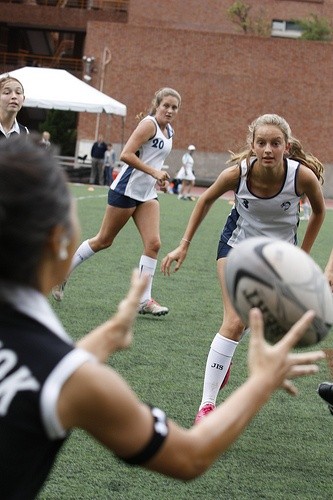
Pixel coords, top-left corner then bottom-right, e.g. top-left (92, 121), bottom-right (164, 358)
top-left (52, 281), bottom-right (66, 302)
top-left (220, 362), bottom-right (233, 392)
top-left (137, 299), bottom-right (169, 316)
top-left (194, 404), bottom-right (216, 425)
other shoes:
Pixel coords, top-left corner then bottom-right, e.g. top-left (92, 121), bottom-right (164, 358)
top-left (178, 194), bottom-right (187, 200)
top-left (318, 382), bottom-right (333, 414)
top-left (187, 194), bottom-right (195, 201)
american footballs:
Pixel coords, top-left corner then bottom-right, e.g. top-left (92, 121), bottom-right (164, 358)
top-left (224, 236), bottom-right (333, 349)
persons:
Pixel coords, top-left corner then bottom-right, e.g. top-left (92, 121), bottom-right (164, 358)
top-left (102, 143), bottom-right (119, 186)
top-left (39, 132), bottom-right (50, 151)
top-left (90, 133), bottom-right (109, 186)
top-left (316, 251), bottom-right (332, 417)
top-left (158, 112), bottom-right (326, 460)
top-left (0, 75), bottom-right (31, 141)
top-left (175, 144), bottom-right (197, 201)
top-left (0, 132), bottom-right (325, 500)
top-left (50, 86), bottom-right (183, 317)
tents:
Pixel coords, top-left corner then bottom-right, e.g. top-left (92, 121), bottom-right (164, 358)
top-left (0, 66), bottom-right (126, 153)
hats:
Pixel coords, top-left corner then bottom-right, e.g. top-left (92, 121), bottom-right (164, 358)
top-left (188, 145), bottom-right (196, 150)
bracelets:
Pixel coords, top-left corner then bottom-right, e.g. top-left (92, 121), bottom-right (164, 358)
top-left (182, 238), bottom-right (190, 244)
